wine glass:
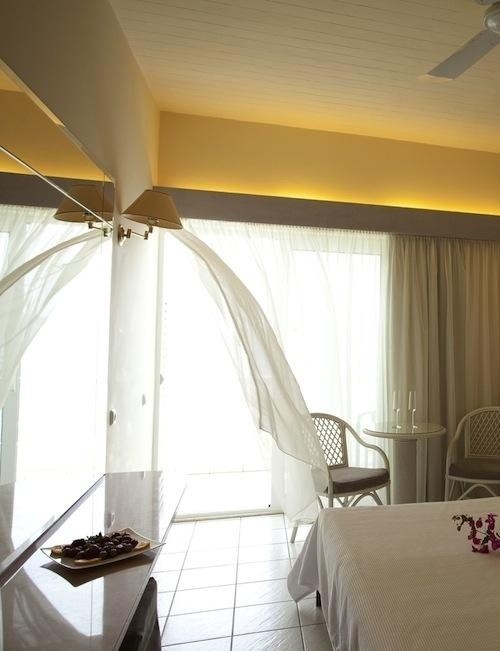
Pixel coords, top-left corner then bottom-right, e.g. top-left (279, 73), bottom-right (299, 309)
top-left (392, 390), bottom-right (403, 429)
top-left (407, 391), bottom-right (419, 428)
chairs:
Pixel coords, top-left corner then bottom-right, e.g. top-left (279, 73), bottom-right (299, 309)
top-left (442, 405), bottom-right (500, 501)
top-left (291, 410), bottom-right (392, 545)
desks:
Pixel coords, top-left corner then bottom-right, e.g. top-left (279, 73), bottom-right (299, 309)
top-left (361, 418), bottom-right (446, 504)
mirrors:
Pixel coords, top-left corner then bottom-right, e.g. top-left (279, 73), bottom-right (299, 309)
top-left (2, 56), bottom-right (110, 586)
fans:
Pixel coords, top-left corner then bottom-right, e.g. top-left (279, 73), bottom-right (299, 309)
top-left (423, 0), bottom-right (500, 81)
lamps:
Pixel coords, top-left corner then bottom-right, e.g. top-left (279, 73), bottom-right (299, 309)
top-left (116, 188), bottom-right (185, 247)
top-left (51, 181), bottom-right (113, 237)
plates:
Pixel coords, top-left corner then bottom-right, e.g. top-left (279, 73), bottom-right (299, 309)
top-left (38, 550), bottom-right (158, 588)
top-left (38, 526), bottom-right (166, 571)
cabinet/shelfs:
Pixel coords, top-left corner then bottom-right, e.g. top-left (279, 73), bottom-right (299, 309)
top-left (0, 467), bottom-right (196, 651)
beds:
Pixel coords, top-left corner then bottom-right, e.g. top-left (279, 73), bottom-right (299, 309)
top-left (285, 494), bottom-right (500, 650)
